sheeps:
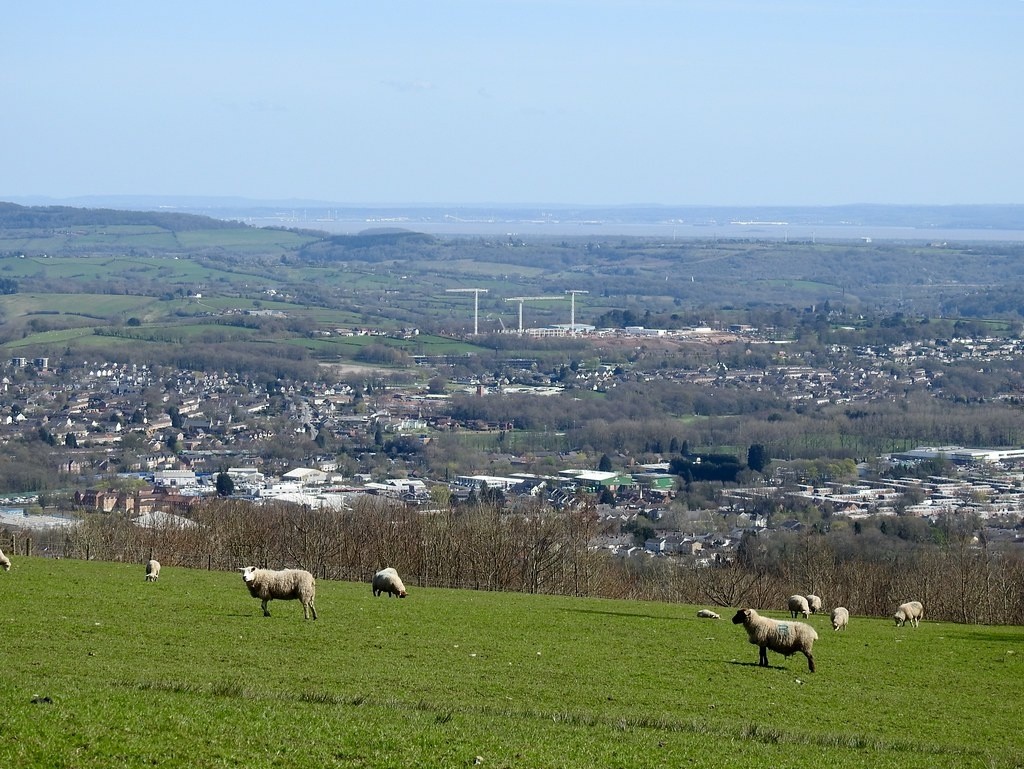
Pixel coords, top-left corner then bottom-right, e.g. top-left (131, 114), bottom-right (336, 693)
top-left (0, 548), bottom-right (11, 572)
top-left (697, 609), bottom-right (721, 619)
top-left (787, 594), bottom-right (821, 619)
top-left (372, 567), bottom-right (409, 598)
top-left (894, 601), bottom-right (924, 629)
top-left (830, 607), bottom-right (849, 632)
top-left (145, 560), bottom-right (161, 582)
top-left (732, 608), bottom-right (819, 673)
top-left (236, 566), bottom-right (318, 620)
